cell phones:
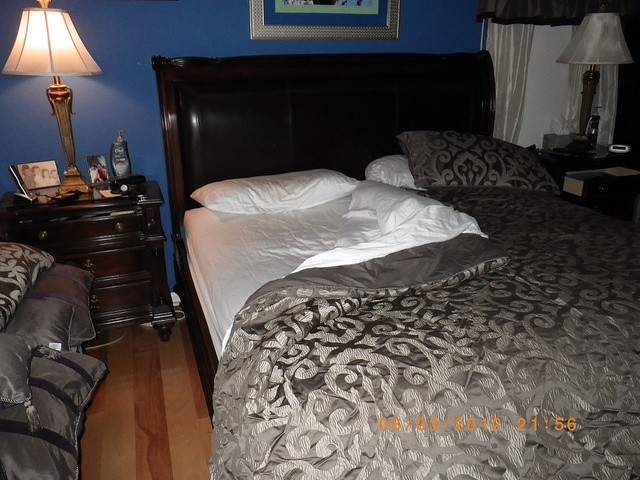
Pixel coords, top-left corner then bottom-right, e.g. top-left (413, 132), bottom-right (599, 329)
top-left (51, 190), bottom-right (82, 201)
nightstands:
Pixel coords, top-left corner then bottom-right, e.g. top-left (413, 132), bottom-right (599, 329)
top-left (0, 181), bottom-right (177, 343)
top-left (535, 146), bottom-right (639, 223)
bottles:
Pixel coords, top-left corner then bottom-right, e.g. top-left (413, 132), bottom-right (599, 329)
top-left (585, 106), bottom-right (602, 151)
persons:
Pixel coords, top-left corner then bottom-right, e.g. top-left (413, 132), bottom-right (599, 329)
top-left (94, 163), bottom-right (109, 184)
top-left (20, 165), bottom-right (60, 189)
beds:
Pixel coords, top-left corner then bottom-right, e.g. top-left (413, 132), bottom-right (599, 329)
top-left (152, 50), bottom-right (639, 480)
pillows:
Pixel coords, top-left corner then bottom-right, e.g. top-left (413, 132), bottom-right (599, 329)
top-left (0, 333), bottom-right (44, 433)
top-left (396, 131), bottom-right (560, 198)
top-left (365, 155), bottom-right (428, 192)
top-left (24, 263), bottom-right (96, 347)
top-left (5, 297), bottom-right (77, 351)
top-left (190, 168), bottom-right (359, 213)
top-left (0, 242), bottom-right (56, 330)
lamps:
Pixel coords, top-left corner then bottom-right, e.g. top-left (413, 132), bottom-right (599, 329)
top-left (1, 1), bottom-right (103, 193)
top-left (553, 13), bottom-right (633, 153)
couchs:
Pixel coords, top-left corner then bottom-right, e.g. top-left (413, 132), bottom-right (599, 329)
top-left (0, 241), bottom-right (108, 480)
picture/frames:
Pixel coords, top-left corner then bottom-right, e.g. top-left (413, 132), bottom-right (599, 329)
top-left (86, 153), bottom-right (110, 186)
top-left (16, 160), bottom-right (61, 191)
top-left (249, 0), bottom-right (400, 42)
